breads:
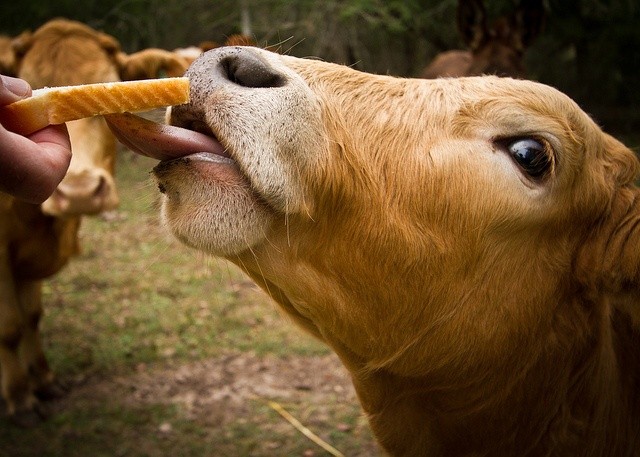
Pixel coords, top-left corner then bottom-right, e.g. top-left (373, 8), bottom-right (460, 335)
top-left (7, 76), bottom-right (192, 136)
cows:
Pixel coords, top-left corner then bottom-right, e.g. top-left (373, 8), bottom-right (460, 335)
top-left (0, 18), bottom-right (194, 429)
top-left (100, 44), bottom-right (640, 457)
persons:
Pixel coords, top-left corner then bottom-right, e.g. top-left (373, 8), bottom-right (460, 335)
top-left (0, 73), bottom-right (73, 205)
